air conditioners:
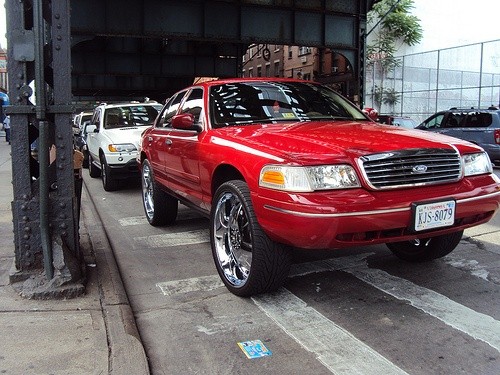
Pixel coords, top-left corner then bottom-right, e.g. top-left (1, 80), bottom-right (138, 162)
top-left (332, 67), bottom-right (338, 72)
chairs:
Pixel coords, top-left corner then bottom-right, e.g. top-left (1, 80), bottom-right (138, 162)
top-left (441, 115), bottom-right (458, 127)
top-left (461, 115), bottom-right (472, 126)
top-left (107, 115), bottom-right (120, 126)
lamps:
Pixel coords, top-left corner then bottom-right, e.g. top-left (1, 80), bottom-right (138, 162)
top-left (162, 31), bottom-right (168, 45)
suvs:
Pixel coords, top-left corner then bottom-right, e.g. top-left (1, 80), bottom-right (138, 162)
top-left (84, 98), bottom-right (165, 193)
top-left (411, 106), bottom-right (500, 169)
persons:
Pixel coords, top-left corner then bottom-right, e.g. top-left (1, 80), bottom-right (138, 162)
top-left (3, 114), bottom-right (11, 145)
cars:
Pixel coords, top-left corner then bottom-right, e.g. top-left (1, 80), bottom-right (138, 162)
top-left (138, 76), bottom-right (500, 296)
top-left (74, 111), bottom-right (96, 168)
